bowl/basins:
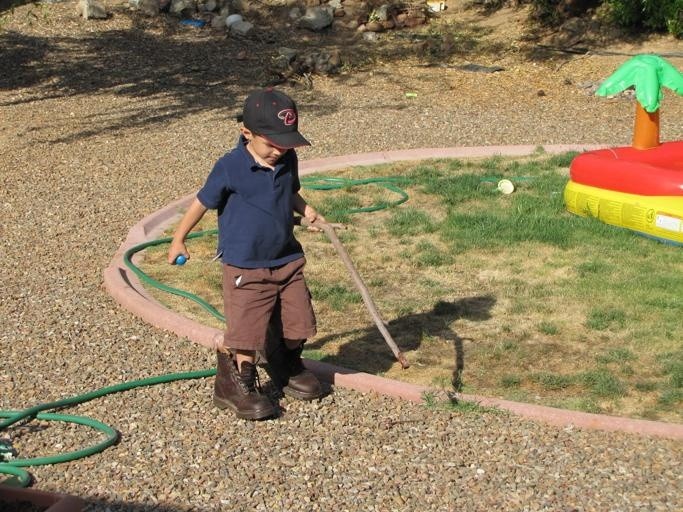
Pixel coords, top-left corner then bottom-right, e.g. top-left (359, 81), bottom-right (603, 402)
top-left (225, 13), bottom-right (242, 29)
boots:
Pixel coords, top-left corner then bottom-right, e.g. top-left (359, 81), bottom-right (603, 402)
top-left (260, 335), bottom-right (321, 399)
top-left (212, 350), bottom-right (274, 419)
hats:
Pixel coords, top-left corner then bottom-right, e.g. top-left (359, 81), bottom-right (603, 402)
top-left (243, 88), bottom-right (311, 149)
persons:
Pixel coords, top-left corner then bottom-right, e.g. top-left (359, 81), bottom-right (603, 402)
top-left (166, 87), bottom-right (329, 420)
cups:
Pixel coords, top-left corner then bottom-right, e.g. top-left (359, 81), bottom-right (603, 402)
top-left (497, 178), bottom-right (515, 195)
